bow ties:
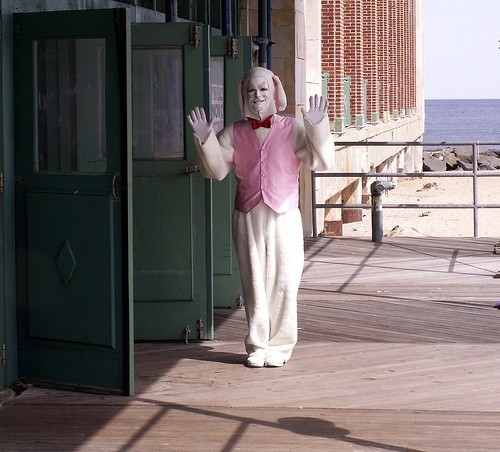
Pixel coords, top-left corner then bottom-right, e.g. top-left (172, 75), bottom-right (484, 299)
top-left (252, 118), bottom-right (272, 129)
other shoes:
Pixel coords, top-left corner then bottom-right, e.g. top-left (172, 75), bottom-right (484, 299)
top-left (265, 356), bottom-right (284, 367)
top-left (247, 356), bottom-right (264, 367)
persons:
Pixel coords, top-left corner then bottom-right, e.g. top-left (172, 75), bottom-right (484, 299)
top-left (186, 67), bottom-right (334, 368)
